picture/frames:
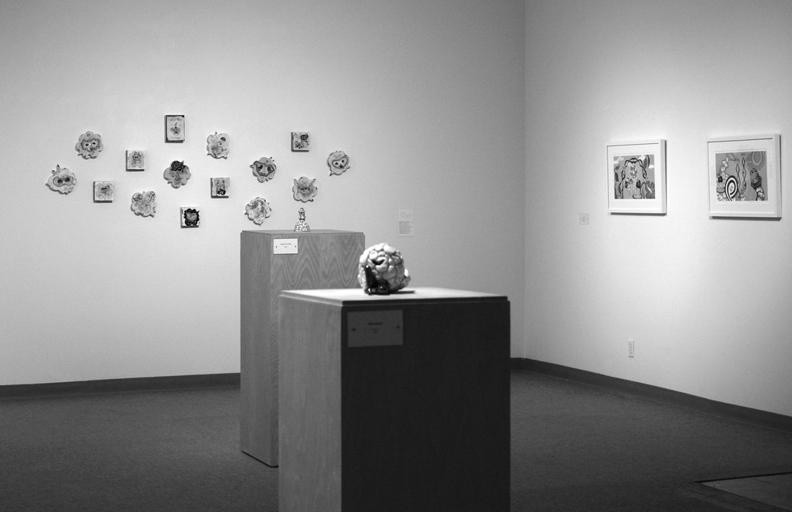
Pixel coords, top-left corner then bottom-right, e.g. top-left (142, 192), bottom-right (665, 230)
top-left (604, 138), bottom-right (667, 216)
top-left (704, 133), bottom-right (780, 218)
